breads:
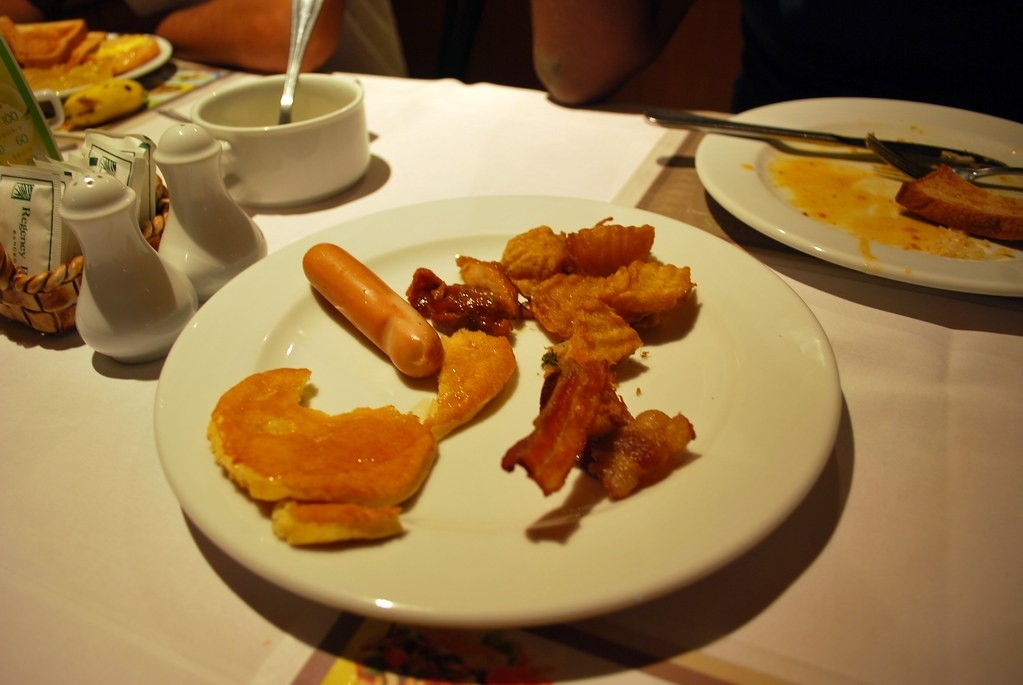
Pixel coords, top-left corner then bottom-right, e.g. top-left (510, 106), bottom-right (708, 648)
top-left (895, 166), bottom-right (1022, 241)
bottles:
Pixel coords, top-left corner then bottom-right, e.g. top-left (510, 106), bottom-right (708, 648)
top-left (57, 173), bottom-right (197, 363)
top-left (148, 122), bottom-right (265, 300)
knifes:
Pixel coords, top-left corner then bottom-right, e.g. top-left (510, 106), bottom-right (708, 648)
top-left (643, 109), bottom-right (1008, 174)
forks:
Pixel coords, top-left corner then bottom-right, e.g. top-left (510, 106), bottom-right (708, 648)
top-left (864, 132), bottom-right (1022, 194)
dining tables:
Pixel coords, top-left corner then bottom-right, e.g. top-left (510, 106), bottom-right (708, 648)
top-left (0, 68), bottom-right (1023, 685)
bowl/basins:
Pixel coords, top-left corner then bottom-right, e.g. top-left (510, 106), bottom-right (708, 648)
top-left (191, 71), bottom-right (371, 211)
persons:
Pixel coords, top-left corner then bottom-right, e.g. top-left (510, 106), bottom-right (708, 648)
top-left (0, 0), bottom-right (414, 82)
top-left (528, 0), bottom-right (1022, 128)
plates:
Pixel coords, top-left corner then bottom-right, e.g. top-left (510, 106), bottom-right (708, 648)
top-left (690, 96), bottom-right (1022, 299)
top-left (28, 31), bottom-right (172, 102)
top-left (152, 196), bottom-right (842, 629)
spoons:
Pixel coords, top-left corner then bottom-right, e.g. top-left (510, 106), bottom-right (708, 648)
top-left (275, 0), bottom-right (328, 126)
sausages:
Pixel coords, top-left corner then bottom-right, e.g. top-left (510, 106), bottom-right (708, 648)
top-left (302, 242), bottom-right (445, 378)
